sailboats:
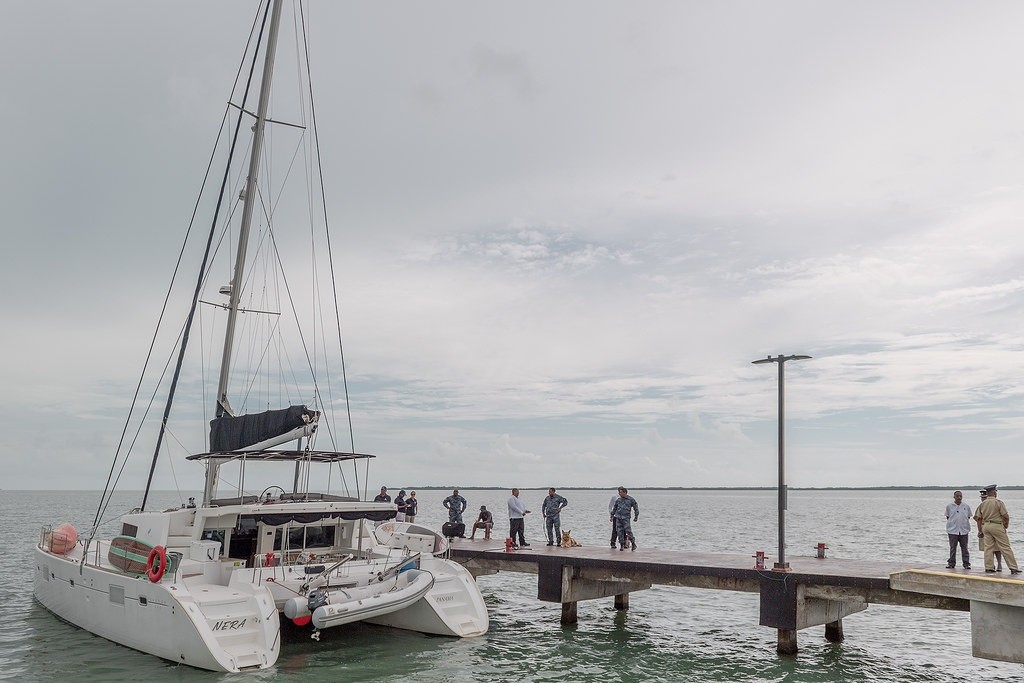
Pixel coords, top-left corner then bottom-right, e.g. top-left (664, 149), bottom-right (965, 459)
top-left (32, 0), bottom-right (490, 673)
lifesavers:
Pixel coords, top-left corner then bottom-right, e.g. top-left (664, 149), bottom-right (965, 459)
top-left (145, 544), bottom-right (167, 584)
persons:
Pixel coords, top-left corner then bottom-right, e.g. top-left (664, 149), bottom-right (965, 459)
top-left (405, 492), bottom-right (418, 523)
top-left (443, 489), bottom-right (466, 538)
top-left (394, 490), bottom-right (412, 522)
top-left (542, 488), bottom-right (568, 547)
top-left (944, 491), bottom-right (972, 569)
top-left (973, 485), bottom-right (1022, 574)
top-left (979, 489), bottom-right (1002, 572)
top-left (374, 486), bottom-right (391, 530)
top-left (609, 486), bottom-right (630, 549)
top-left (468, 505), bottom-right (494, 539)
top-left (508, 488), bottom-right (532, 547)
top-left (610, 488), bottom-right (639, 550)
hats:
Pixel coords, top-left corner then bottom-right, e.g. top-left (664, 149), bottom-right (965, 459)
top-left (399, 490), bottom-right (407, 496)
top-left (980, 491), bottom-right (987, 497)
top-left (618, 486), bottom-right (625, 491)
top-left (453, 489), bottom-right (459, 493)
top-left (983, 485), bottom-right (997, 491)
top-left (479, 505), bottom-right (486, 510)
top-left (381, 486), bottom-right (388, 492)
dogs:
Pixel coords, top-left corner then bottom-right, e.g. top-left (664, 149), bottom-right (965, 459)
top-left (561, 530), bottom-right (582, 548)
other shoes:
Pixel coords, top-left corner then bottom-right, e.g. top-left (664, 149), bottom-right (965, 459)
top-left (631, 542), bottom-right (637, 551)
top-left (459, 534), bottom-right (466, 538)
top-left (547, 542), bottom-right (553, 546)
top-left (611, 545), bottom-right (617, 549)
top-left (557, 543), bottom-right (561, 546)
top-left (946, 564), bottom-right (954, 568)
top-left (985, 569), bottom-right (996, 573)
top-left (964, 566), bottom-right (971, 569)
top-left (994, 566), bottom-right (995, 570)
top-left (1011, 570), bottom-right (1022, 574)
top-left (511, 543), bottom-right (518, 548)
top-left (520, 541), bottom-right (530, 546)
top-left (624, 543), bottom-right (629, 549)
top-left (620, 545), bottom-right (624, 551)
top-left (996, 569), bottom-right (1002, 572)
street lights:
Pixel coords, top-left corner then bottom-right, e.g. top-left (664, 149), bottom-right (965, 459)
top-left (751, 354), bottom-right (812, 572)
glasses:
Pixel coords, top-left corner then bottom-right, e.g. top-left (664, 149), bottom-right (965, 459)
top-left (411, 494), bottom-right (415, 496)
top-left (481, 510), bottom-right (483, 511)
top-left (454, 491), bottom-right (457, 492)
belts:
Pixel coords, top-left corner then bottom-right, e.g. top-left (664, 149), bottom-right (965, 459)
top-left (984, 521), bottom-right (1004, 524)
